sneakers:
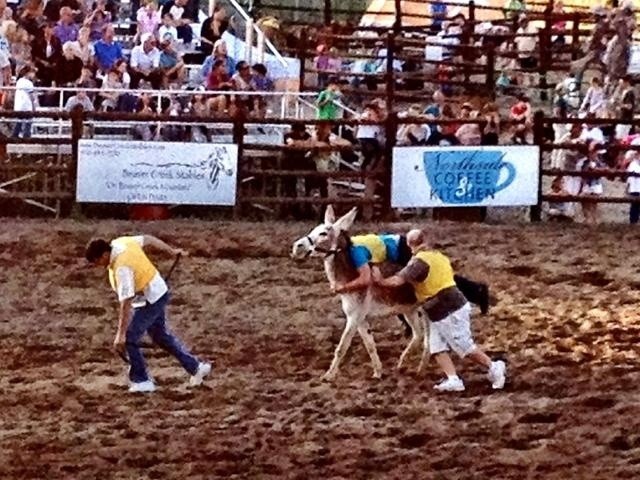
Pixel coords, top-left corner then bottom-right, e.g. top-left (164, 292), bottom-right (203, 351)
top-left (480, 282), bottom-right (489, 316)
top-left (188, 361), bottom-right (212, 388)
top-left (128, 379), bottom-right (155, 393)
top-left (434, 378), bottom-right (466, 394)
top-left (488, 359), bottom-right (506, 389)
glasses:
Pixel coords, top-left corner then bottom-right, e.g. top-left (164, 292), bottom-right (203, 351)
top-left (241, 65), bottom-right (249, 71)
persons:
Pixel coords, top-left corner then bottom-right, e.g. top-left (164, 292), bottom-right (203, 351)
top-left (370, 228), bottom-right (507, 396)
top-left (314, 0), bottom-right (639, 228)
top-left (327, 232), bottom-right (491, 318)
top-left (281, 118), bottom-right (350, 198)
top-left (0, 0), bottom-right (271, 136)
top-left (83, 233), bottom-right (213, 395)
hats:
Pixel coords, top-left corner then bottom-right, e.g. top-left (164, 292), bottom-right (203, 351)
top-left (37, 15), bottom-right (60, 29)
top-left (25, 63), bottom-right (38, 71)
top-left (326, 75), bottom-right (341, 86)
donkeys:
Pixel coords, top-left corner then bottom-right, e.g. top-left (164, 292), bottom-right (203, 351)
top-left (293, 205), bottom-right (430, 382)
